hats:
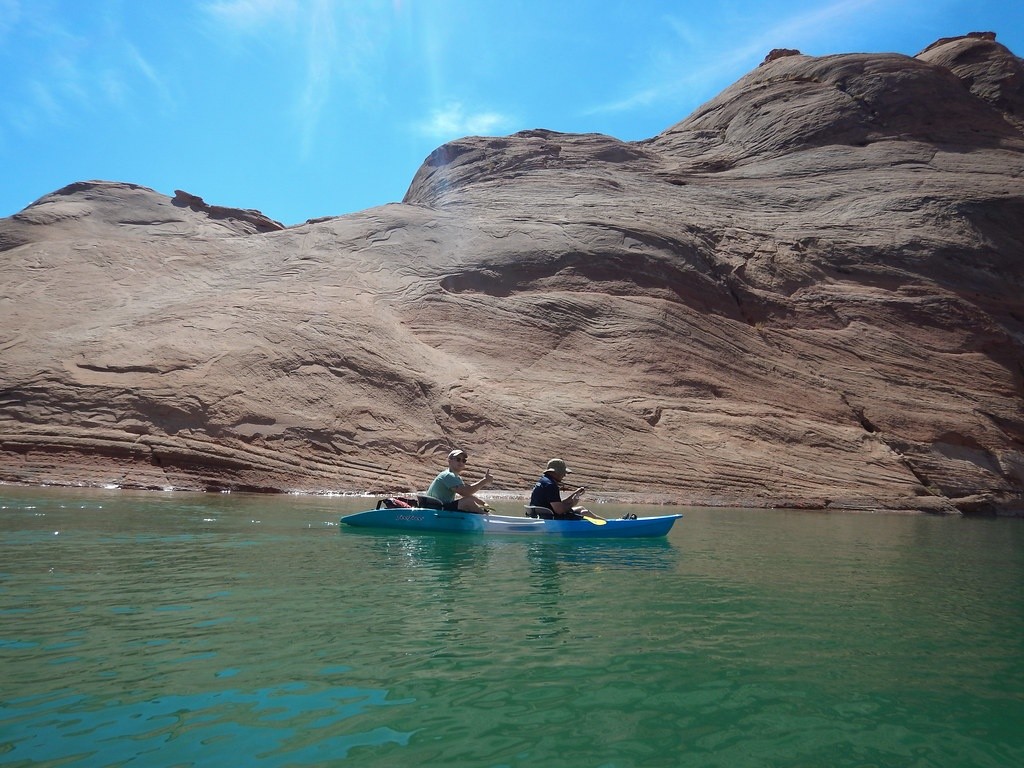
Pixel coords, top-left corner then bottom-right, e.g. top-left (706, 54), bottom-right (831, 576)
top-left (448, 449), bottom-right (468, 458)
top-left (543, 459), bottom-right (572, 473)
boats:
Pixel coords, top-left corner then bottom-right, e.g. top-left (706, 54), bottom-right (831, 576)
top-left (338, 505), bottom-right (683, 539)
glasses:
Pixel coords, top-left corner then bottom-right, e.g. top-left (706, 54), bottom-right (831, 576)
top-left (452, 458), bottom-right (467, 464)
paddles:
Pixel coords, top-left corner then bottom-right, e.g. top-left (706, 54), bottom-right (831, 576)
top-left (570, 510), bottom-right (607, 526)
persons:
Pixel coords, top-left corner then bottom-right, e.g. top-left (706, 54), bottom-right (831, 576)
top-left (427, 449), bottom-right (493, 515)
top-left (528, 459), bottom-right (605, 520)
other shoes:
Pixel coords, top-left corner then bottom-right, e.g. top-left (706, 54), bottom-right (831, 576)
top-left (630, 514), bottom-right (637, 521)
top-left (618, 512), bottom-right (629, 519)
